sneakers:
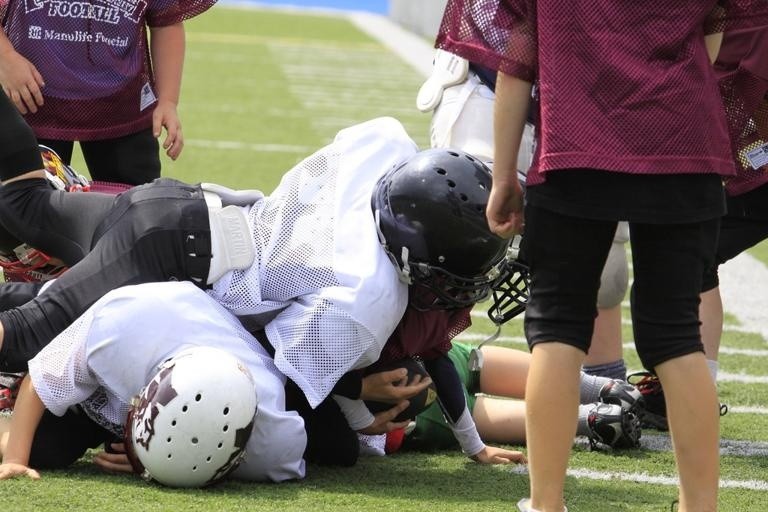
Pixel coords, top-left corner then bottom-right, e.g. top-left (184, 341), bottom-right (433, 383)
top-left (587, 373), bottom-right (669, 449)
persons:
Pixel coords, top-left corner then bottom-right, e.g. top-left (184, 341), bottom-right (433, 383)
top-left (416, 1), bottom-right (629, 383)
top-left (641, 0), bottom-right (767, 430)
top-left (487, 0), bottom-right (740, 512)
top-left (0, 84), bottom-right (529, 479)
top-left (0, 0), bottom-right (218, 185)
top-left (337, 340), bottom-right (647, 455)
top-left (1, 280), bottom-right (310, 488)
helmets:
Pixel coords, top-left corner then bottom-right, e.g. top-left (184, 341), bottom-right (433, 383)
top-left (125, 344), bottom-right (256, 487)
top-left (371, 149), bottom-right (534, 325)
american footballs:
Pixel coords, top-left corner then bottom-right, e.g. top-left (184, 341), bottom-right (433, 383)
top-left (360, 359), bottom-right (437, 423)
